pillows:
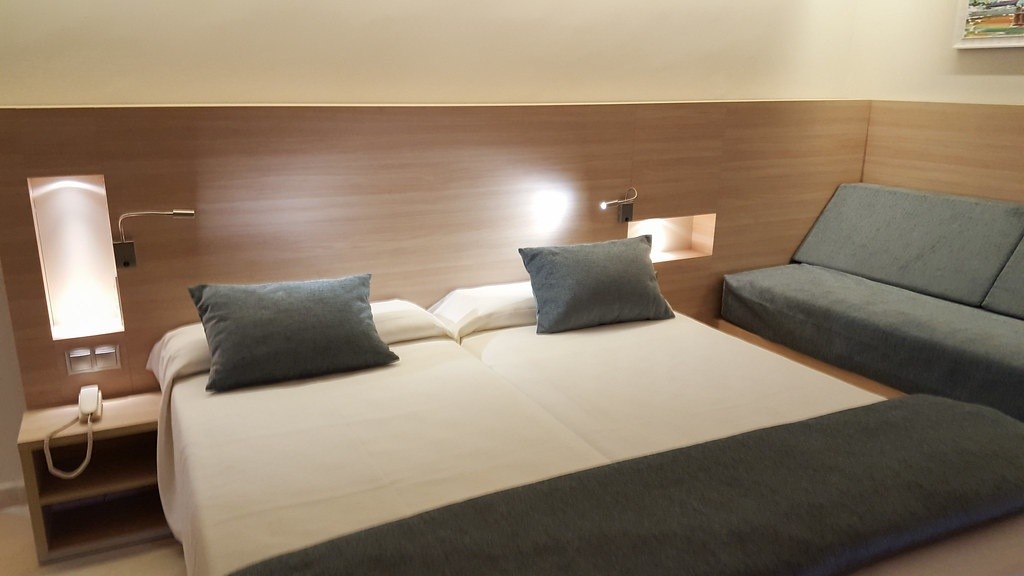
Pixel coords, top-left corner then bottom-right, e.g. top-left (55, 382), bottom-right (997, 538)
top-left (518, 233), bottom-right (676, 334)
top-left (188, 273), bottom-right (399, 393)
top-left (431, 282), bottom-right (537, 341)
top-left (159, 299), bottom-right (447, 376)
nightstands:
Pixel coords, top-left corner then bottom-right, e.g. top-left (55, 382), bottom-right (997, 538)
top-left (16, 388), bottom-right (173, 566)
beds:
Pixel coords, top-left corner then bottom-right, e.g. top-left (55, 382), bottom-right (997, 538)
top-left (157, 311), bottom-right (1024, 576)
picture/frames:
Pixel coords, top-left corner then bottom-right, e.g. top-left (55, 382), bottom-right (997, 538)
top-left (951, 0), bottom-right (1024, 50)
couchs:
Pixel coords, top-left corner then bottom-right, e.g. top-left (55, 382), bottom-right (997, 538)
top-left (721, 181), bottom-right (1024, 422)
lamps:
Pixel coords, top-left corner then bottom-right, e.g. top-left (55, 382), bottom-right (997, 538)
top-left (112, 211), bottom-right (195, 267)
top-left (602, 187), bottom-right (637, 224)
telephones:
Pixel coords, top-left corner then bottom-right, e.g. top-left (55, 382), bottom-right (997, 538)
top-left (77, 384), bottom-right (103, 422)
